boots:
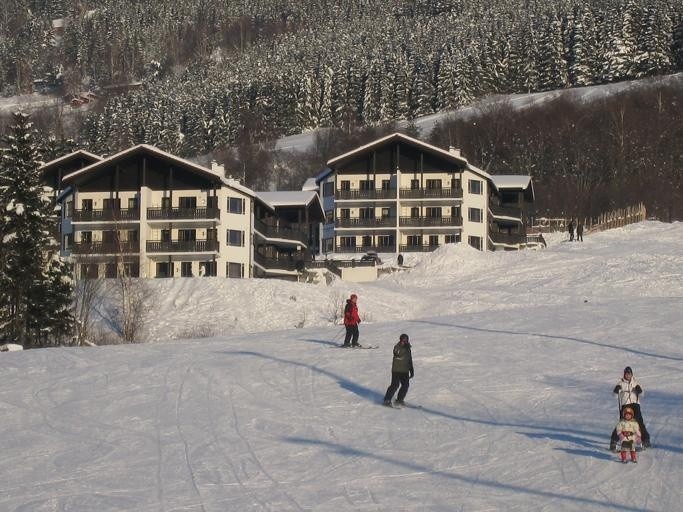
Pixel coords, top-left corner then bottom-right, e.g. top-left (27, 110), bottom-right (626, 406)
top-left (610, 445), bottom-right (617, 453)
top-left (343, 342), bottom-right (362, 348)
top-left (384, 399), bottom-right (405, 408)
top-left (642, 439), bottom-right (651, 447)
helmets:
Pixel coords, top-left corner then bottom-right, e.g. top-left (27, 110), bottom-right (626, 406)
top-left (623, 407), bottom-right (634, 417)
top-left (624, 367), bottom-right (632, 373)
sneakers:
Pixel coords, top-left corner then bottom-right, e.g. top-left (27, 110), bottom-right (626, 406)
top-left (622, 459), bottom-right (637, 464)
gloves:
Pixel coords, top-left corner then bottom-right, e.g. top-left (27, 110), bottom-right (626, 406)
top-left (635, 385), bottom-right (642, 393)
top-left (357, 319), bottom-right (361, 324)
top-left (349, 317), bottom-right (353, 320)
top-left (409, 370), bottom-right (414, 379)
top-left (618, 433), bottom-right (627, 441)
top-left (613, 385), bottom-right (621, 394)
top-left (405, 343), bottom-right (412, 347)
top-left (635, 436), bottom-right (642, 446)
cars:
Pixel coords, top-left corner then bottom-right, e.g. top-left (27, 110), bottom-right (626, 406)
top-left (359, 251), bottom-right (382, 265)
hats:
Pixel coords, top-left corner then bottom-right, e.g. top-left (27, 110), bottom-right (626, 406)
top-left (351, 294), bottom-right (357, 298)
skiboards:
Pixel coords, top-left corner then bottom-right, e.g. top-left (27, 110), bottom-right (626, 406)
top-left (381, 400), bottom-right (422, 411)
top-left (328, 343), bottom-right (379, 349)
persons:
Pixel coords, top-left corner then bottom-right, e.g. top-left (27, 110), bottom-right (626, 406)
top-left (576, 223), bottom-right (583, 241)
top-left (384, 334), bottom-right (414, 408)
top-left (610, 367), bottom-right (651, 464)
top-left (398, 254), bottom-right (403, 266)
top-left (568, 219), bottom-right (575, 241)
top-left (344, 294), bottom-right (361, 347)
